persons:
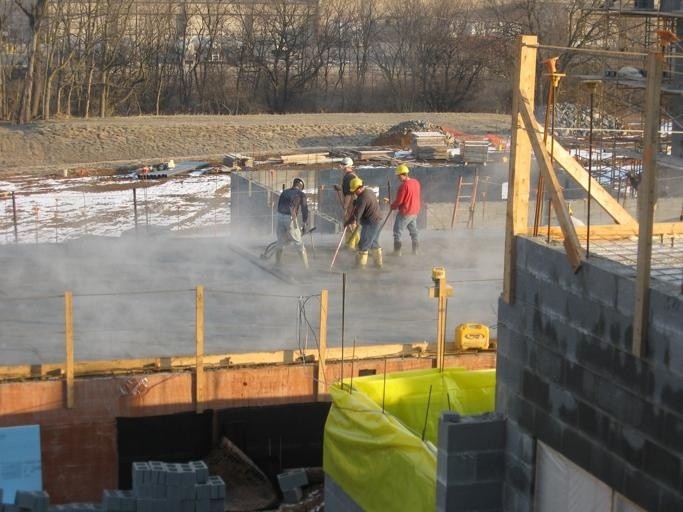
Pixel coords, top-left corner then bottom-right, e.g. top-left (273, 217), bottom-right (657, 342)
top-left (333, 157), bottom-right (359, 250)
top-left (387, 165), bottom-right (421, 257)
top-left (275, 177), bottom-right (309, 270)
top-left (343, 178), bottom-right (383, 269)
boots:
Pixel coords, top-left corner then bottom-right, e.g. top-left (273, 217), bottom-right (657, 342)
top-left (411, 240), bottom-right (419, 255)
top-left (387, 240), bottom-right (401, 256)
top-left (299, 249), bottom-right (309, 269)
top-left (350, 251), bottom-right (368, 270)
top-left (371, 248), bottom-right (383, 269)
top-left (275, 248), bottom-right (285, 264)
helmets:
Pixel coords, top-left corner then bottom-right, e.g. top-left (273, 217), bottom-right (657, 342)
top-left (349, 177), bottom-right (363, 191)
top-left (340, 157), bottom-right (353, 169)
top-left (395, 165), bottom-right (409, 176)
top-left (293, 178), bottom-right (305, 190)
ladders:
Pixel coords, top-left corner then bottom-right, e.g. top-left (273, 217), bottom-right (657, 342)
top-left (451, 176), bottom-right (478, 232)
